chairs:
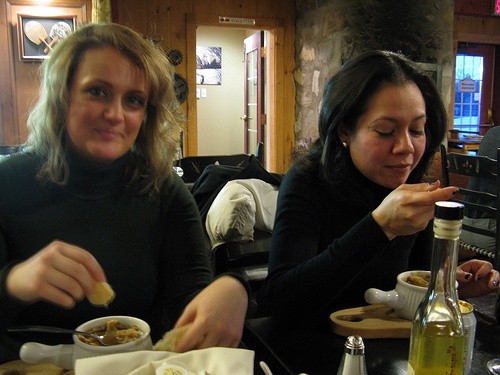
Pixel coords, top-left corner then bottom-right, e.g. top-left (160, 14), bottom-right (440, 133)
top-left (205, 164), bottom-right (287, 298)
top-left (440, 144), bottom-right (500, 273)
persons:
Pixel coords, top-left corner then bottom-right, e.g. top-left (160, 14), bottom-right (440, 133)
top-left (459, 124), bottom-right (500, 257)
top-left (264, 49), bottom-right (500, 319)
top-left (0, 23), bottom-right (253, 353)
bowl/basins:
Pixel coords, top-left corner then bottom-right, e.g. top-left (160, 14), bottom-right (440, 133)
top-left (20, 314), bottom-right (154, 371)
top-left (363, 268), bottom-right (459, 319)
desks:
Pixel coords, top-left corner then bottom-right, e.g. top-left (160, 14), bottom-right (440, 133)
top-left (241, 289), bottom-right (500, 375)
top-left (447, 138), bottom-right (482, 167)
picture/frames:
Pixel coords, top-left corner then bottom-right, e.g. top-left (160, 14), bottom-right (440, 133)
top-left (16, 12), bottom-right (77, 62)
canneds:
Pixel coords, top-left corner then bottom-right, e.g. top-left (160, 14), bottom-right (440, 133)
top-left (427, 297), bottom-right (476, 375)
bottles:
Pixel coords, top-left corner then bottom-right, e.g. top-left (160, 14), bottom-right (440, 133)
top-left (407, 201), bottom-right (469, 375)
top-left (335, 337), bottom-right (369, 375)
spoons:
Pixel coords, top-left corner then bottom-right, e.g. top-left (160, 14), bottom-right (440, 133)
top-left (7, 324), bottom-right (118, 346)
